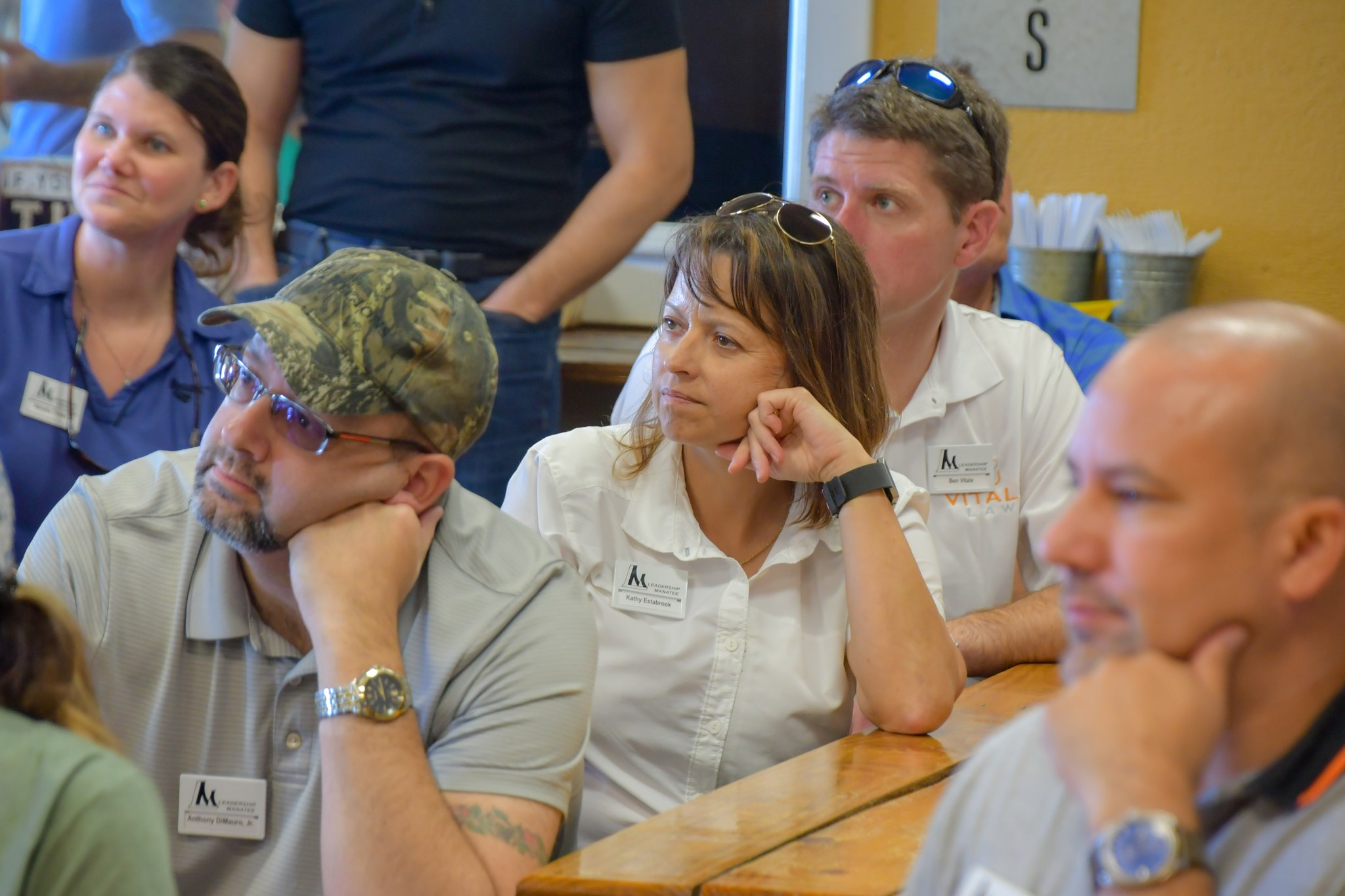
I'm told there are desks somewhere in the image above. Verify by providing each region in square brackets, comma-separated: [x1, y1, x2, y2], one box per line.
[517, 664, 1075, 896]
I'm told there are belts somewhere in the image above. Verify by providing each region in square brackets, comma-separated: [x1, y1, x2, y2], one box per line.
[375, 243, 528, 283]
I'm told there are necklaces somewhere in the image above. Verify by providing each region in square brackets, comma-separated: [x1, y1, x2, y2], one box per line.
[73, 258, 175, 387]
[738, 481, 795, 568]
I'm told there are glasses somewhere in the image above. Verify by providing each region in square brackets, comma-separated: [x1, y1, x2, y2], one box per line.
[208, 340, 432, 457]
[833, 57, 988, 147]
[715, 190, 842, 298]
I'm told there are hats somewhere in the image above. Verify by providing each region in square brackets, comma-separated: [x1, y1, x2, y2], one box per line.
[195, 241, 502, 465]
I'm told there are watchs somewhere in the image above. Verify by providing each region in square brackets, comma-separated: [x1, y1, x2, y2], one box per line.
[315, 665, 415, 724]
[821, 455, 899, 519]
[1087, 807, 1214, 893]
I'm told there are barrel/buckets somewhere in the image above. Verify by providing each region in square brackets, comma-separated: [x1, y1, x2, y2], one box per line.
[1099, 247, 1200, 326]
[998, 245, 1097, 302]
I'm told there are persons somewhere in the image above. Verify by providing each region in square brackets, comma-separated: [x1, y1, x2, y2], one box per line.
[0, 0, 234, 281]
[2, 543, 182, 896]
[610, 57, 1101, 733]
[496, 190, 970, 847]
[1, 42, 256, 592]
[214, 0, 694, 519]
[12, 246, 600, 896]
[889, 299, 1345, 895]
